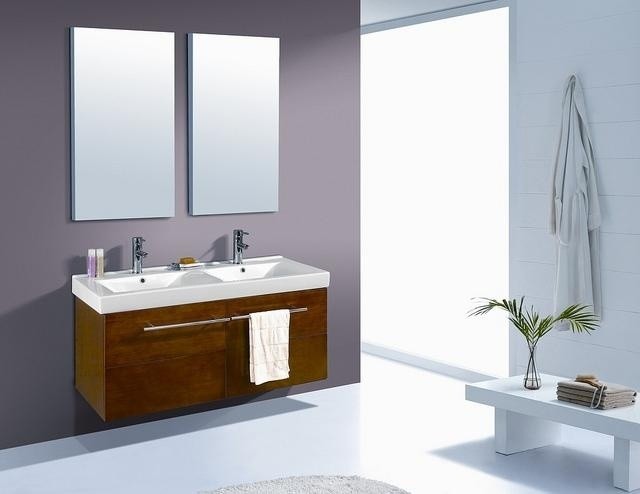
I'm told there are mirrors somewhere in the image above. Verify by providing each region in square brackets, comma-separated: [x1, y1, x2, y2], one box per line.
[188, 32, 280, 217]
[70, 27, 176, 222]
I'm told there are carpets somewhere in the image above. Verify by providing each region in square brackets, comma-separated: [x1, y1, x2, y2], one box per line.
[196, 475, 411, 494]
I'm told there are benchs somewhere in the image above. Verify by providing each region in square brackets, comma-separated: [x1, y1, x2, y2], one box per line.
[465, 373, 640, 492]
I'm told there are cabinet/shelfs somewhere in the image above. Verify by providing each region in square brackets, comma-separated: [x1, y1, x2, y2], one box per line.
[226, 287, 327, 398]
[104, 300, 231, 420]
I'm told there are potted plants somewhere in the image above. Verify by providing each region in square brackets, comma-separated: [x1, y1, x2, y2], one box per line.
[466, 296, 600, 390]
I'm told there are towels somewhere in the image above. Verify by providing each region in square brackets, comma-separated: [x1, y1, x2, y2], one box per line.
[248, 309, 290, 387]
[556, 381, 638, 410]
